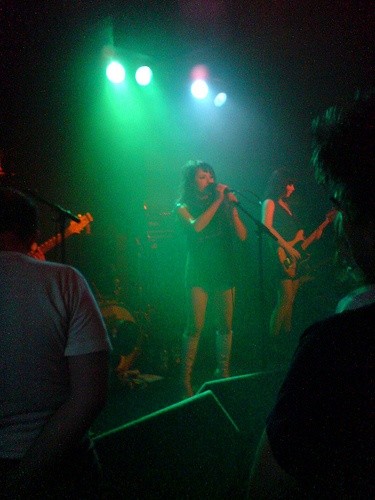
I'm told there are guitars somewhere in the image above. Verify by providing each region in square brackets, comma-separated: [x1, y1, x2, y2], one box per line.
[275, 208, 337, 278]
[25, 212, 93, 262]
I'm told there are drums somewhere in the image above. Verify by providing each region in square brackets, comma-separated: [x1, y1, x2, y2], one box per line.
[97, 303, 138, 373]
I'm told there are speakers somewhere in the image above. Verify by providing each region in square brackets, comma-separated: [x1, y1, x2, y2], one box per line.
[195, 369, 289, 450]
[90, 390, 255, 500]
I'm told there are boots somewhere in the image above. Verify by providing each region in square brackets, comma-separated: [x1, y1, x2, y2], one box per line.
[216, 331, 234, 387]
[179, 333, 197, 405]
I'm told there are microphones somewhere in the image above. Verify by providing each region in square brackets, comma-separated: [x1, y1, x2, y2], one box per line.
[206, 183, 233, 194]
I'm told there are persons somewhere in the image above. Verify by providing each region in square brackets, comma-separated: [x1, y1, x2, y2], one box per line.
[0, 184, 110, 500]
[175, 159, 247, 400]
[245, 88, 375, 500]
[261, 170, 323, 362]
